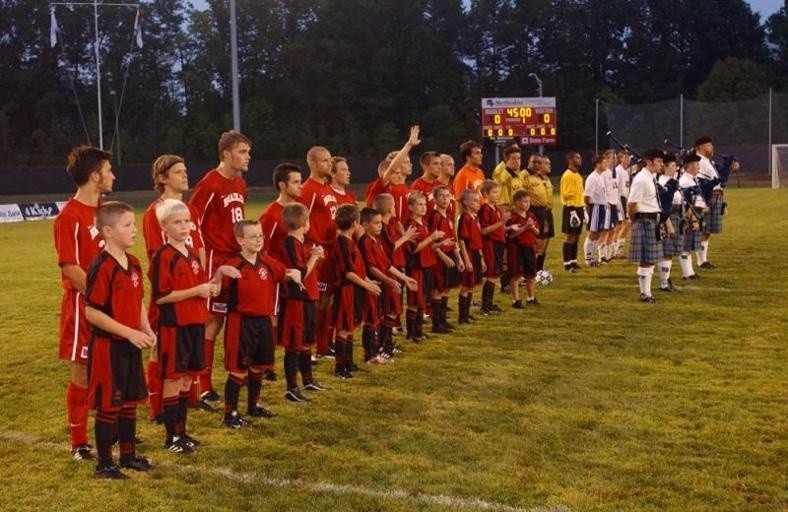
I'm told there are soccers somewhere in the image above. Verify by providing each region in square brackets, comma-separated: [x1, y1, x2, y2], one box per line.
[536, 270, 553, 285]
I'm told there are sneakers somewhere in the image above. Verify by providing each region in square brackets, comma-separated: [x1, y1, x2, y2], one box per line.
[222, 410, 257, 432]
[311, 276, 541, 378]
[283, 387, 312, 404]
[200, 391, 221, 403]
[189, 401, 218, 413]
[94, 463, 130, 482]
[262, 371, 277, 383]
[163, 435, 193, 455]
[247, 406, 277, 418]
[179, 434, 200, 449]
[118, 455, 152, 472]
[565, 254, 715, 305]
[303, 380, 330, 393]
[68, 444, 96, 464]
[149, 415, 166, 427]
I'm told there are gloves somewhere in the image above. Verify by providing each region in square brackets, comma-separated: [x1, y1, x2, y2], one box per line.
[583, 208, 590, 225]
[569, 210, 582, 228]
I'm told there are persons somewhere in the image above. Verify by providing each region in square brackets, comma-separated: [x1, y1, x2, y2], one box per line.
[559, 136, 740, 301]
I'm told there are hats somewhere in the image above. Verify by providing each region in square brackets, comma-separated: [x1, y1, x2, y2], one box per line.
[694, 136, 712, 147]
[640, 150, 677, 163]
[683, 154, 701, 163]
